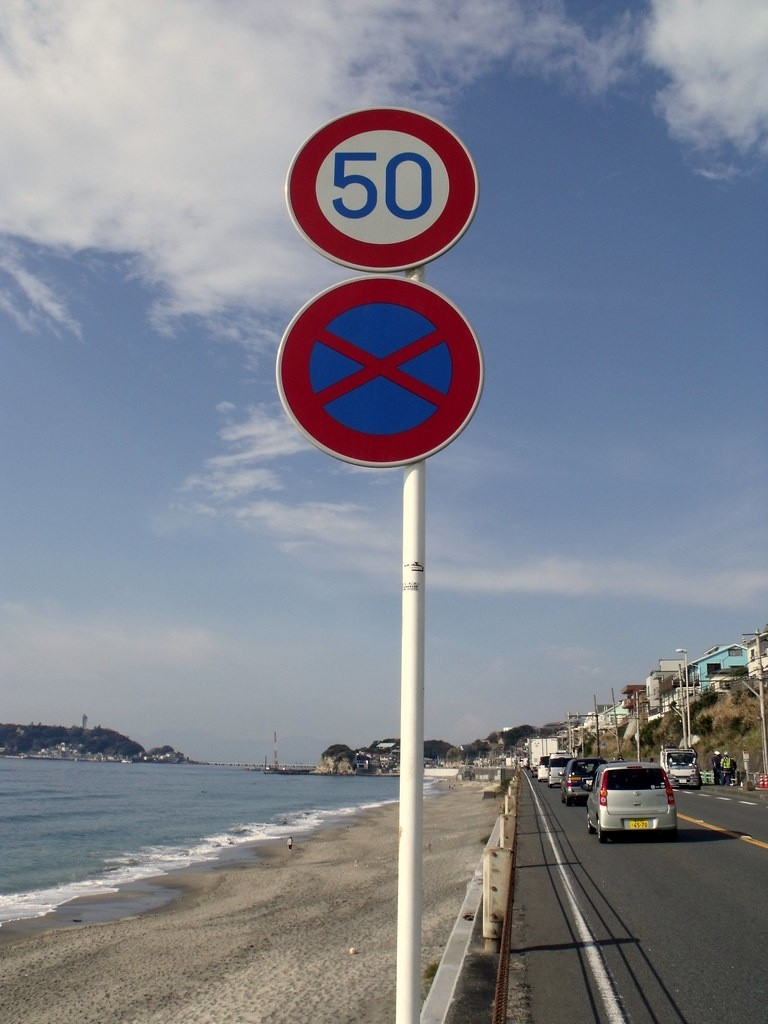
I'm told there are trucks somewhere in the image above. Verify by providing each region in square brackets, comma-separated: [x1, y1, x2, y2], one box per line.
[529, 736, 559, 778]
[660, 747, 701, 790]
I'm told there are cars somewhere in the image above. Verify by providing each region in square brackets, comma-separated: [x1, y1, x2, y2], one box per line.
[581, 762, 678, 844]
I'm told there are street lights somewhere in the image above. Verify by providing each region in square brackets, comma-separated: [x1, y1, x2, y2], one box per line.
[674, 648, 694, 749]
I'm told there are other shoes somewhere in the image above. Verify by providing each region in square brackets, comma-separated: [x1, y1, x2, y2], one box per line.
[714, 783, 721, 785]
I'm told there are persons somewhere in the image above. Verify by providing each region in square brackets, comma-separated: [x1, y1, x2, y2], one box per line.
[711, 751, 737, 786]
[649, 758, 654, 763]
[617, 755, 624, 761]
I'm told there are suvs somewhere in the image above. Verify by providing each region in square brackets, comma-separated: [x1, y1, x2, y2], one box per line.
[548, 751, 574, 789]
[557, 757, 608, 806]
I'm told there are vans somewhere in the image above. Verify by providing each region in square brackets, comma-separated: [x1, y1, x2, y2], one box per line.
[538, 756, 550, 782]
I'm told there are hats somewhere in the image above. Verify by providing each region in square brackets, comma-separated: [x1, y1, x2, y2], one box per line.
[723, 751, 728, 755]
[714, 750, 720, 755]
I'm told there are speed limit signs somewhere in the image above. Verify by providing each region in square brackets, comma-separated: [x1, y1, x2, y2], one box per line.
[285, 105, 482, 275]
